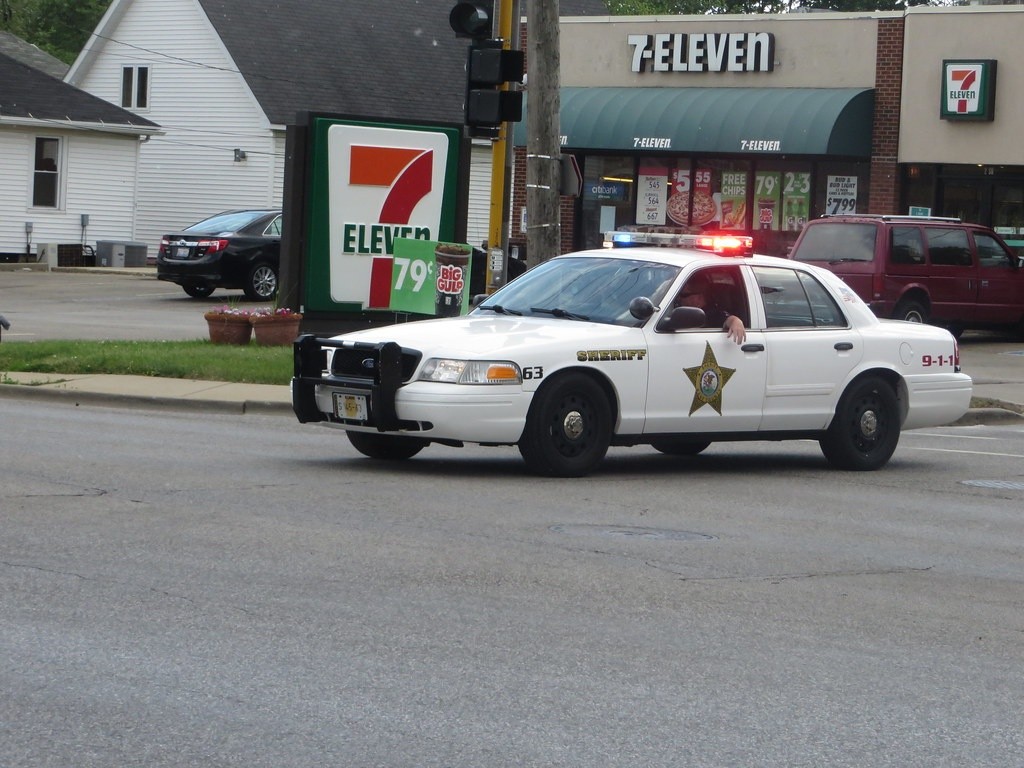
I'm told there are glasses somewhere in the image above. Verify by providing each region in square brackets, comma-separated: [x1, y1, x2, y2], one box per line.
[679, 292, 702, 297]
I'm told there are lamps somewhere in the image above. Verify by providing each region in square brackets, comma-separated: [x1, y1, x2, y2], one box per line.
[233, 149, 246, 161]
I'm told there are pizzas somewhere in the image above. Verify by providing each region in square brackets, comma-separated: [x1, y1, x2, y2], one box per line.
[668, 190, 716, 224]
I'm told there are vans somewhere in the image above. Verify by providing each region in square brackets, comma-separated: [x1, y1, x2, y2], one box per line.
[788, 212, 1024, 343]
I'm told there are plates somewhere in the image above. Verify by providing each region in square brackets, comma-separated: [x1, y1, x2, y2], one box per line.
[666, 211, 716, 226]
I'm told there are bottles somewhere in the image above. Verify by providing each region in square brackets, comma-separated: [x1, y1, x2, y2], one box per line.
[786, 200, 795, 230]
[758, 201, 775, 231]
[797, 199, 807, 231]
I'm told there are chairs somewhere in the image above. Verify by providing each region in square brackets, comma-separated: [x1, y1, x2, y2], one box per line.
[710, 283, 748, 329]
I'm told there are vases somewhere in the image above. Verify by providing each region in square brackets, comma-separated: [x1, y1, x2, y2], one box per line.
[249, 314, 303, 347]
[204, 312, 252, 344]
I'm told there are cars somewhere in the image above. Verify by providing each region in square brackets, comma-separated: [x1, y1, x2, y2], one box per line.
[288, 233, 974, 479]
[157, 207, 282, 302]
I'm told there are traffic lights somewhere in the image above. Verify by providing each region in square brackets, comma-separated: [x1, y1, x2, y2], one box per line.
[447, 0, 494, 42]
[466, 39, 525, 140]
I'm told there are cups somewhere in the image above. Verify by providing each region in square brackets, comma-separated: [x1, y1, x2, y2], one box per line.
[722, 199, 733, 223]
[434, 251, 470, 318]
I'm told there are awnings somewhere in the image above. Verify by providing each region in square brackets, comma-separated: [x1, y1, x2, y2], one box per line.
[512, 84, 874, 161]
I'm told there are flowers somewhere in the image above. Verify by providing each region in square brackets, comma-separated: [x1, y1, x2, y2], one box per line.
[208, 290, 247, 315]
[249, 289, 296, 317]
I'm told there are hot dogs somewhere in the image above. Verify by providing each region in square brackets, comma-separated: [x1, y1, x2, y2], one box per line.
[735, 203, 746, 224]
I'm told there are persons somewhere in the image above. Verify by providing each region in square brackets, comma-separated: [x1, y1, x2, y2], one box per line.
[669, 268, 749, 345]
